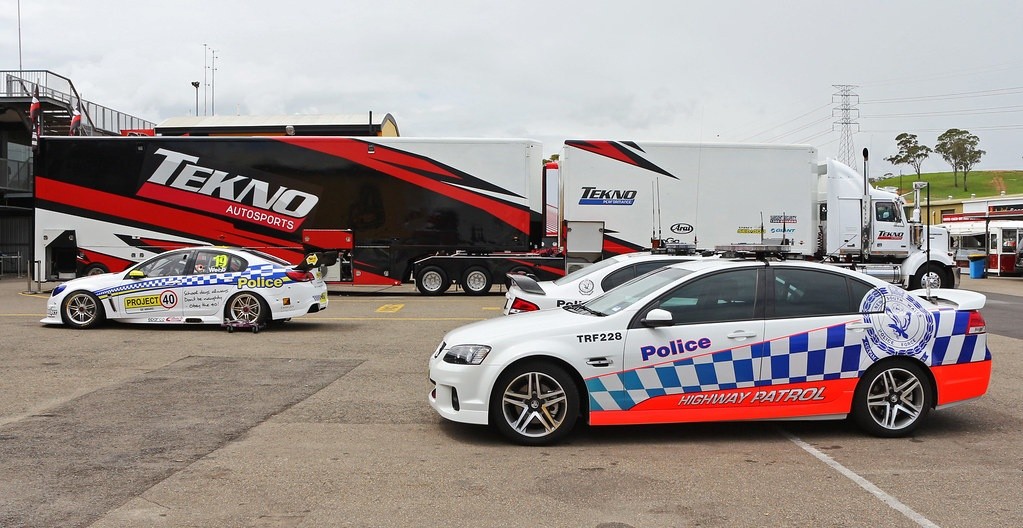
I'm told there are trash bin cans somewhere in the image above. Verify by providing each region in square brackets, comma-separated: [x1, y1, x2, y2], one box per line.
[966, 253, 989, 279]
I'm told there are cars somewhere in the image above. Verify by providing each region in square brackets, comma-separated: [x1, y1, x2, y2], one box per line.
[502, 246, 805, 321]
[424, 240, 991, 446]
[42, 241, 338, 328]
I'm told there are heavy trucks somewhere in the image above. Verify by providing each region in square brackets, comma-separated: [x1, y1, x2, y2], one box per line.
[28, 129, 562, 294]
[554, 139, 958, 293]
